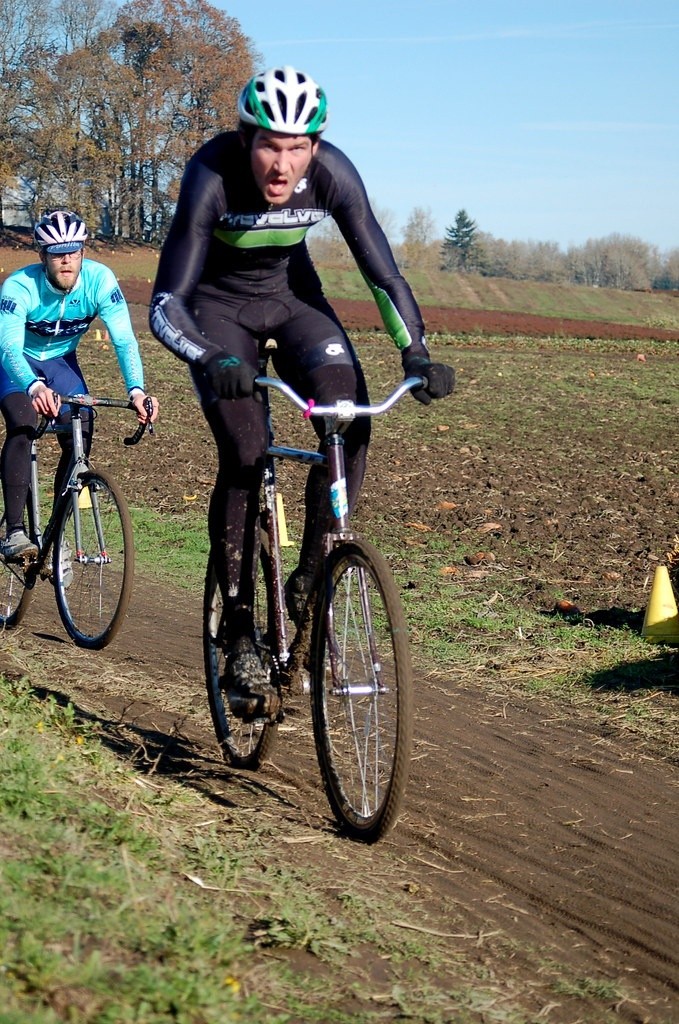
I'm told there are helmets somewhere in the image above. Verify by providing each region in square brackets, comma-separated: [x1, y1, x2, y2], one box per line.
[33, 209, 89, 253]
[237, 67, 328, 135]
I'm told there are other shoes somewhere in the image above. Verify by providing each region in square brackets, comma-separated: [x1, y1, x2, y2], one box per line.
[222, 639, 280, 718]
[286, 567, 316, 625]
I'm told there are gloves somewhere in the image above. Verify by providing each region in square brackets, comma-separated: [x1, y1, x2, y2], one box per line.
[199, 346, 258, 402]
[400, 354, 454, 405]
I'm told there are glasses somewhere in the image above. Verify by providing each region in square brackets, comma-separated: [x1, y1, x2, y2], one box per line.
[48, 251, 82, 261]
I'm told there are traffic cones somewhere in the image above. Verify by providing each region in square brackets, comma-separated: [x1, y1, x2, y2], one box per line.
[639, 566, 679, 642]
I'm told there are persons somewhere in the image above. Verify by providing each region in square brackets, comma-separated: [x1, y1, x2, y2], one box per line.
[147, 65, 454, 718]
[0, 212, 159, 562]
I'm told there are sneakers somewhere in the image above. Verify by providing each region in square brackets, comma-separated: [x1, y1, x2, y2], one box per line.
[46, 540, 74, 588]
[0, 529, 38, 558]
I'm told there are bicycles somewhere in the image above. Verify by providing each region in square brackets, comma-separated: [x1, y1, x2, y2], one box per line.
[205, 339, 429, 847]
[0, 377, 158, 651]
